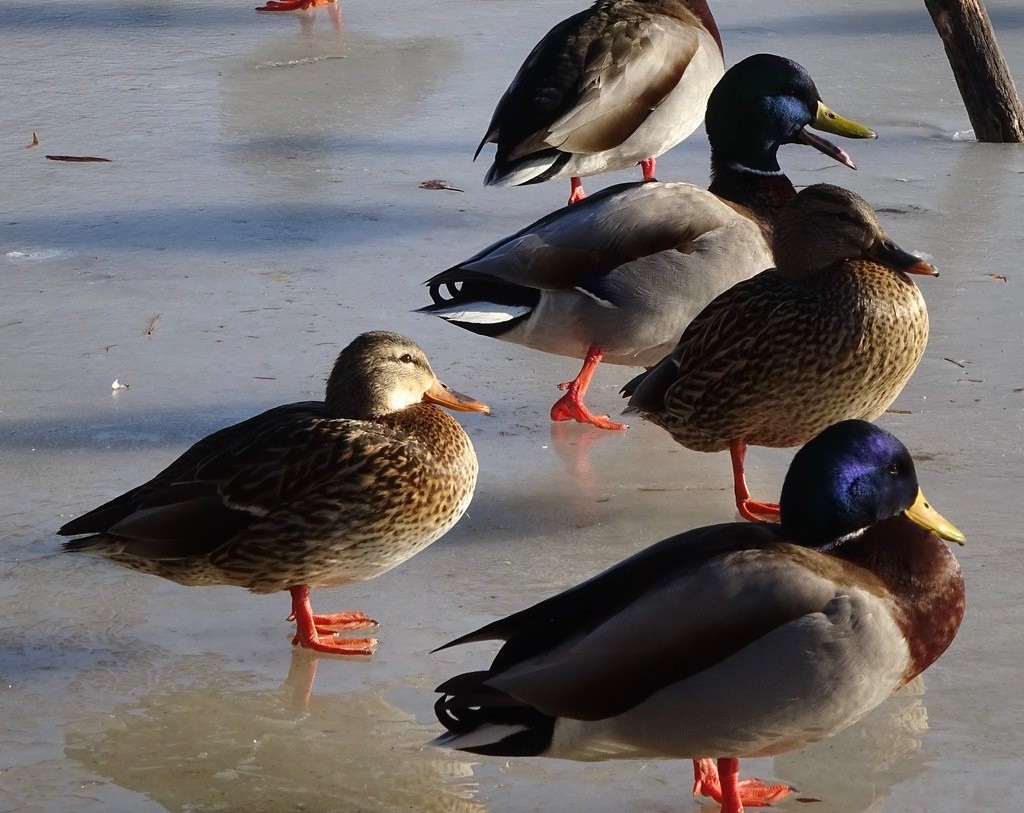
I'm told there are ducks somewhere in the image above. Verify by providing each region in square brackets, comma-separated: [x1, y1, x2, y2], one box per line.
[56, 329, 491, 660]
[408, 1, 968, 813]
